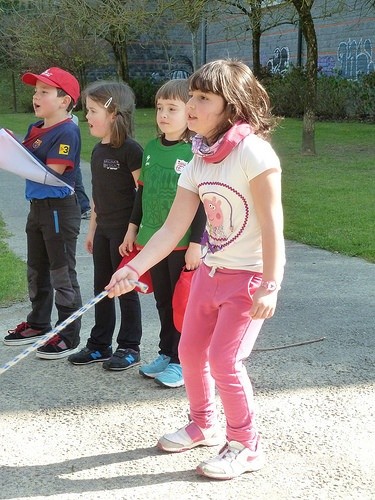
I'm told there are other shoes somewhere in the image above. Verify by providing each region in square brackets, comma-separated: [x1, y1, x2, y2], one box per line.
[81, 209, 93, 220]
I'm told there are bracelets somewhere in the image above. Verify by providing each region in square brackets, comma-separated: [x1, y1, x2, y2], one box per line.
[125, 264, 140, 278]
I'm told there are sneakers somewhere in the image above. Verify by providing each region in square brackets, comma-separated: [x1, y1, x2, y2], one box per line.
[154, 363, 185, 387]
[138, 354, 171, 379]
[36, 334, 80, 359]
[67, 344, 113, 365]
[156, 422, 222, 453]
[101, 347, 142, 371]
[2, 322, 53, 346]
[195, 440, 265, 480]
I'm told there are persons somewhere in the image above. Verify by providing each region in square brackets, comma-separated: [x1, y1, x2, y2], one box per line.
[68, 82, 145, 370]
[2, 66, 91, 359]
[104, 60, 285, 480]
[119, 79, 208, 388]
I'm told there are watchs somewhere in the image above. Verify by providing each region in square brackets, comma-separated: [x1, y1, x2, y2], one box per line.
[258, 279, 282, 291]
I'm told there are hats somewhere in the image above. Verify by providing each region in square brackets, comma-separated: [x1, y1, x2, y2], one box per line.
[21, 67, 81, 107]
[117, 247, 155, 294]
[171, 265, 197, 334]
